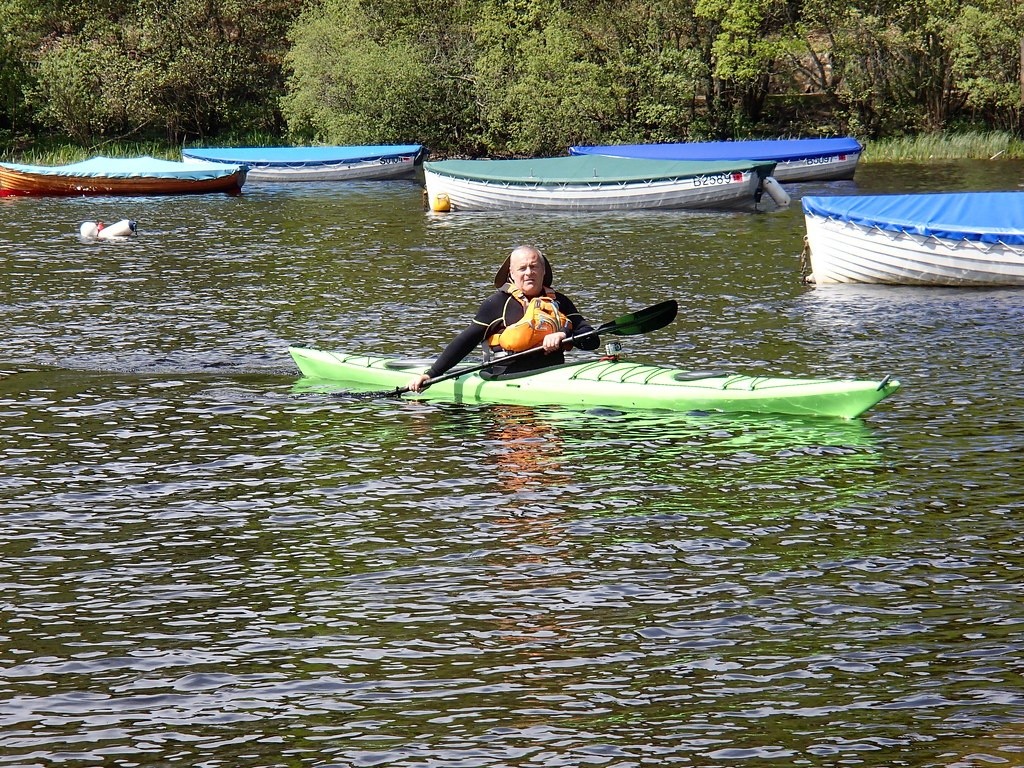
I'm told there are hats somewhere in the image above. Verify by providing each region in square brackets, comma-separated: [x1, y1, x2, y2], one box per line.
[494, 252, 553, 289]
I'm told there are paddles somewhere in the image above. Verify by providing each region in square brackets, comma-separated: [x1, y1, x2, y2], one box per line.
[335, 299, 678, 400]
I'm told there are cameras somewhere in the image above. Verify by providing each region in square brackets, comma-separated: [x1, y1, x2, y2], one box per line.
[605, 341, 623, 356]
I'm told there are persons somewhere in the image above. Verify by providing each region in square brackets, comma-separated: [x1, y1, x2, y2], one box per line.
[406, 244, 600, 392]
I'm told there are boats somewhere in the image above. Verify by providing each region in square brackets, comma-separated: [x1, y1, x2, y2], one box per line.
[570, 138, 863, 185]
[0, 156, 250, 197]
[287, 346, 901, 420]
[423, 155, 791, 211]
[182, 145, 425, 182]
[802, 192, 1024, 287]
